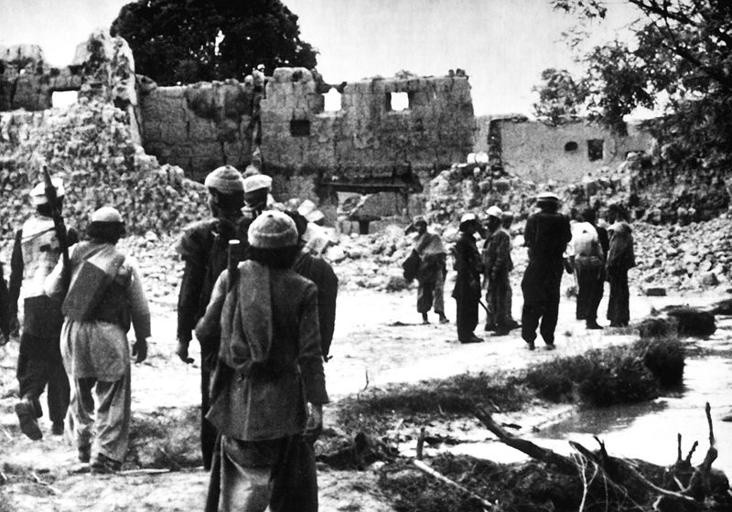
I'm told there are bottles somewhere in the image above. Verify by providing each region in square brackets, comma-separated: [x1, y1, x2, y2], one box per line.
[412, 192, 562, 224]
[244, 175, 273, 193]
[206, 165, 245, 191]
[30, 177, 65, 205]
[93, 207, 124, 222]
[247, 211, 298, 248]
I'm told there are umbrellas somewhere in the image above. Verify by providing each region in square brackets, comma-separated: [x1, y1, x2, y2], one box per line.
[15, 401, 120, 473]
[459, 322, 555, 350]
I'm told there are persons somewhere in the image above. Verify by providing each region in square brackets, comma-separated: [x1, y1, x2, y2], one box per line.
[2, 178, 78, 441]
[562, 205, 636, 329]
[402, 217, 450, 324]
[45, 206, 151, 473]
[478, 206, 520, 336]
[450, 212, 485, 344]
[175, 165, 339, 509]
[522, 191, 572, 350]
